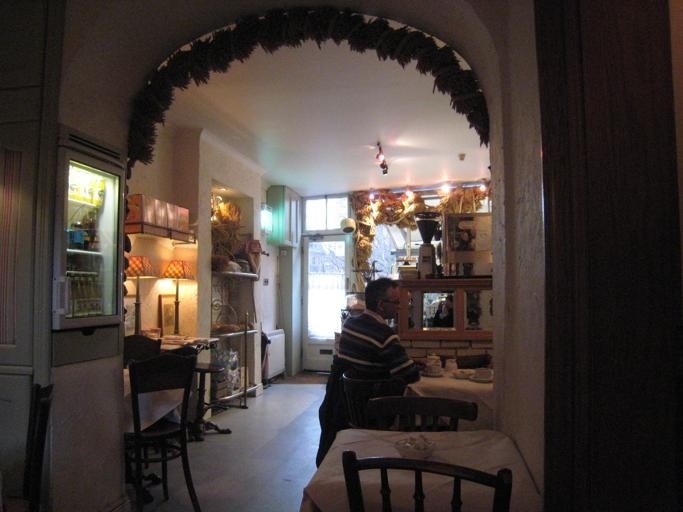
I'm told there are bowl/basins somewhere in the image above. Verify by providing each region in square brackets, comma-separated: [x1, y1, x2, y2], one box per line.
[395, 438, 436, 462]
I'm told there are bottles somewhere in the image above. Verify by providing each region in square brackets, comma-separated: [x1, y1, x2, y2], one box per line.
[64, 270, 104, 320]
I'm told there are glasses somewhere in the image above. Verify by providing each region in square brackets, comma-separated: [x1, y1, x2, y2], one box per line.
[383, 299, 399, 305]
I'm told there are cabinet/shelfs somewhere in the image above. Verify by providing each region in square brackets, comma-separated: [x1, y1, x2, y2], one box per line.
[126, 193, 196, 246]
[266, 186, 302, 248]
[211, 270, 264, 401]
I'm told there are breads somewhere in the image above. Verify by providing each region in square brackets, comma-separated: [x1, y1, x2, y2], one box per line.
[211, 322, 254, 332]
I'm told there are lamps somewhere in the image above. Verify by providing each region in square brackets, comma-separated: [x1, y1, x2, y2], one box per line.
[125, 255, 197, 337]
[340, 195, 356, 235]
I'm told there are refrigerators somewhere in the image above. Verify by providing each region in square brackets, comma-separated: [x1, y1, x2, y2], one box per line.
[52, 121, 124, 334]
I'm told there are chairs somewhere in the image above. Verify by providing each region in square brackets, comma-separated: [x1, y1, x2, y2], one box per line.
[304, 354, 540, 511]
[123, 334, 231, 512]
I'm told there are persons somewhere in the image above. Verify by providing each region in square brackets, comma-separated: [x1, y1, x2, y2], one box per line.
[316, 278, 422, 469]
[432, 295, 454, 327]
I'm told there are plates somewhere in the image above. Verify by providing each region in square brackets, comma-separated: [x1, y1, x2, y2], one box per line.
[421, 366, 494, 382]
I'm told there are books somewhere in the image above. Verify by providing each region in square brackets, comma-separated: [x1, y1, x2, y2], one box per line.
[398, 265, 420, 281]
[162, 335, 209, 346]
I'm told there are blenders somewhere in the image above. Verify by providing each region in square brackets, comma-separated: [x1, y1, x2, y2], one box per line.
[414, 211, 438, 281]
